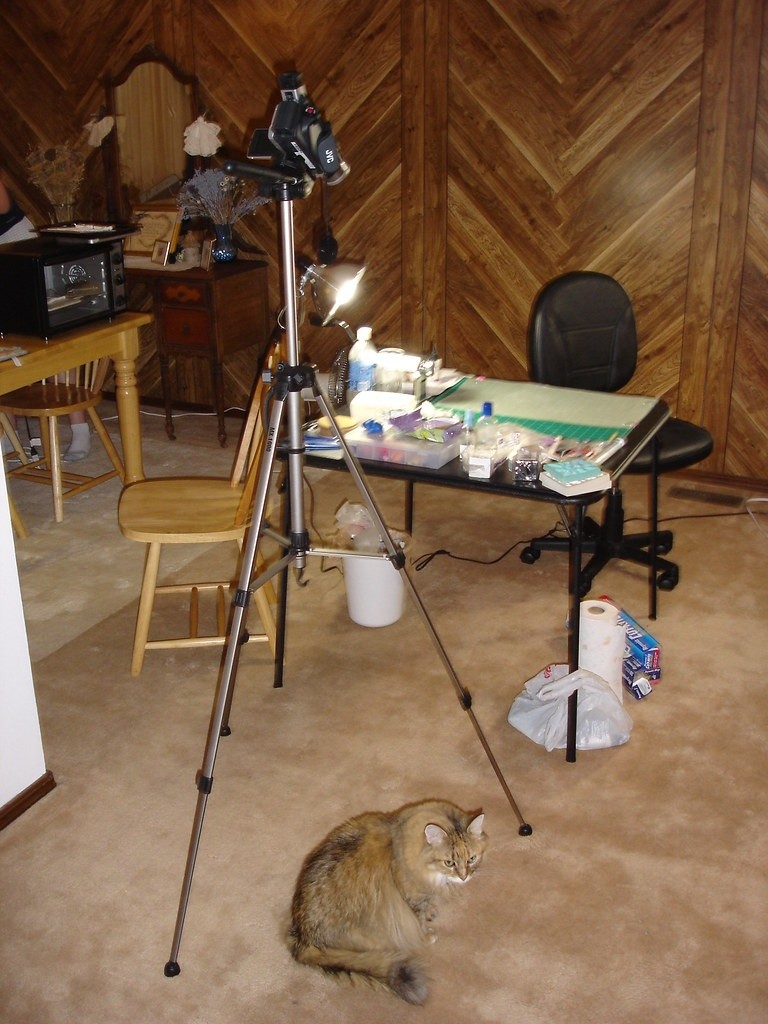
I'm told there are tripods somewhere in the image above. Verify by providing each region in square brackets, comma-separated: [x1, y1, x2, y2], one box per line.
[162, 158, 531, 979]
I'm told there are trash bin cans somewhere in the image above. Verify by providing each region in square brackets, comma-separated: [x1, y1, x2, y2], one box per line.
[332, 529, 415, 627]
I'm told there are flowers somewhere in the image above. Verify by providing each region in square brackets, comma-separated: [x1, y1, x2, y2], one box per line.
[176, 167, 271, 224]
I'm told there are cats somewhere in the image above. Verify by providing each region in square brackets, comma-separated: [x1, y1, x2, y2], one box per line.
[285, 797, 490, 1008]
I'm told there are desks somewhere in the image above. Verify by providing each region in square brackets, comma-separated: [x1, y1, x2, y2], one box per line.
[0, 313, 157, 486]
[273, 371, 671, 763]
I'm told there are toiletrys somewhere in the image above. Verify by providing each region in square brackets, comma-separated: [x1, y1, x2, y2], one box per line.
[594, 596, 663, 702]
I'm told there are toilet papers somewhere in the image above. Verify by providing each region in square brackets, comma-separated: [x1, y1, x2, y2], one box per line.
[578, 600, 627, 708]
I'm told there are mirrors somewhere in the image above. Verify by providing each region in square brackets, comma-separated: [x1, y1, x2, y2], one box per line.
[104, 44, 199, 226]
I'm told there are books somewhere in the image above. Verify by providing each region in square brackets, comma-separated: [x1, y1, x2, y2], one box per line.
[539, 459, 611, 496]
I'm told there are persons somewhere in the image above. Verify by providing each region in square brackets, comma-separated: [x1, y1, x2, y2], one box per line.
[0, 180, 91, 460]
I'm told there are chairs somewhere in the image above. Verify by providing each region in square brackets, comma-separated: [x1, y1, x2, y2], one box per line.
[118, 334, 280, 675]
[0, 357, 125, 523]
[520, 271, 712, 595]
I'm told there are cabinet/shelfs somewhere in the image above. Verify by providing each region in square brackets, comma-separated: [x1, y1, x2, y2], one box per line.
[101, 259, 269, 449]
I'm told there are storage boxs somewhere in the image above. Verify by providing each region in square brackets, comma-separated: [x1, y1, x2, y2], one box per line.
[344, 419, 468, 469]
[514, 460, 538, 481]
[460, 432, 520, 478]
[598, 595, 663, 700]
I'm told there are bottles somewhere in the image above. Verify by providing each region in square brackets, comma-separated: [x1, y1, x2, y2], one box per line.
[474, 402, 499, 459]
[348, 327, 377, 403]
[459, 408, 474, 461]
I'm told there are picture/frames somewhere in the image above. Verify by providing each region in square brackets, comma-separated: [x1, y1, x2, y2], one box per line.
[200, 238, 213, 271]
[122, 205, 185, 257]
[150, 239, 170, 267]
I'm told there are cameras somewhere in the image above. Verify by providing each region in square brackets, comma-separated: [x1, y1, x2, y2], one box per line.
[269, 70, 350, 186]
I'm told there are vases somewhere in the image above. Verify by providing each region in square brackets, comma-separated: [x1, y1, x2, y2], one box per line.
[50, 200, 78, 223]
[212, 224, 237, 264]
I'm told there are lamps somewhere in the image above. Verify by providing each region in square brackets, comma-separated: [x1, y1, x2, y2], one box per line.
[295, 252, 370, 342]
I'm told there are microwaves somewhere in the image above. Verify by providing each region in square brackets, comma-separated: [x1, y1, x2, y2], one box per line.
[0, 237, 127, 340]
